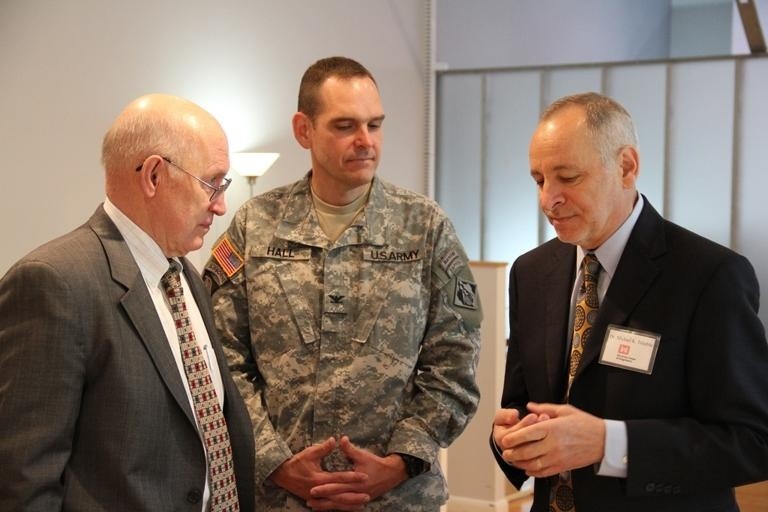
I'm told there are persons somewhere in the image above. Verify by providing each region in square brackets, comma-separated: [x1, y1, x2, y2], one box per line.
[488, 91, 768, 512]
[201, 55, 480, 512]
[0, 90, 257, 512]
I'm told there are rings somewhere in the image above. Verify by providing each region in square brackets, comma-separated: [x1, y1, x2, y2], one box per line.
[535, 458, 542, 472]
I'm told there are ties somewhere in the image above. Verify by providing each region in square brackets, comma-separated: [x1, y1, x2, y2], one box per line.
[548, 253, 601, 511]
[162, 267, 242, 512]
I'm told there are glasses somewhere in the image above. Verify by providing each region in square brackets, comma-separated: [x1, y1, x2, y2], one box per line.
[135, 156, 233, 203]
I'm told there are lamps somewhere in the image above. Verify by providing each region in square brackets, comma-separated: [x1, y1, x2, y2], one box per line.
[230, 153, 279, 199]
